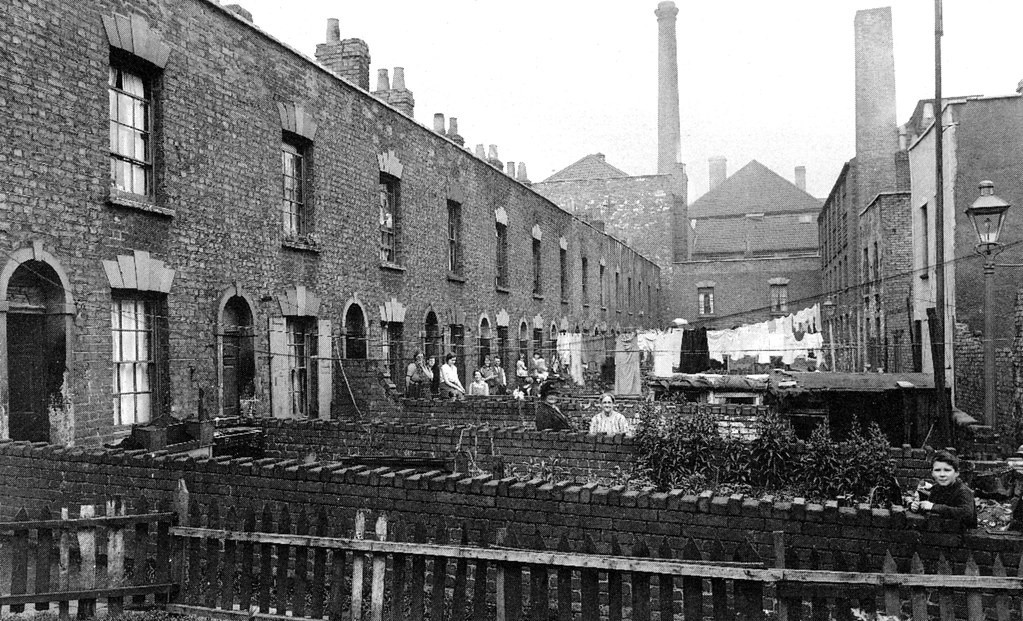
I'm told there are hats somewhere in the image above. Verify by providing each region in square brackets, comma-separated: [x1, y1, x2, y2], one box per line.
[540, 380, 560, 396]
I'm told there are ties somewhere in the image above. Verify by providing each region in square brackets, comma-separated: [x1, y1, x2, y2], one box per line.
[496, 366, 499, 374]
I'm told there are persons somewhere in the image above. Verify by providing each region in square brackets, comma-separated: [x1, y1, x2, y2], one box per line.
[589, 393, 629, 436]
[535, 381, 573, 430]
[406, 350, 587, 401]
[707, 367, 729, 375]
[905, 451, 978, 530]
[640, 346, 654, 367]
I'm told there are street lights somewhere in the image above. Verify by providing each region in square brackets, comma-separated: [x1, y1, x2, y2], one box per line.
[962, 178, 1014, 453]
